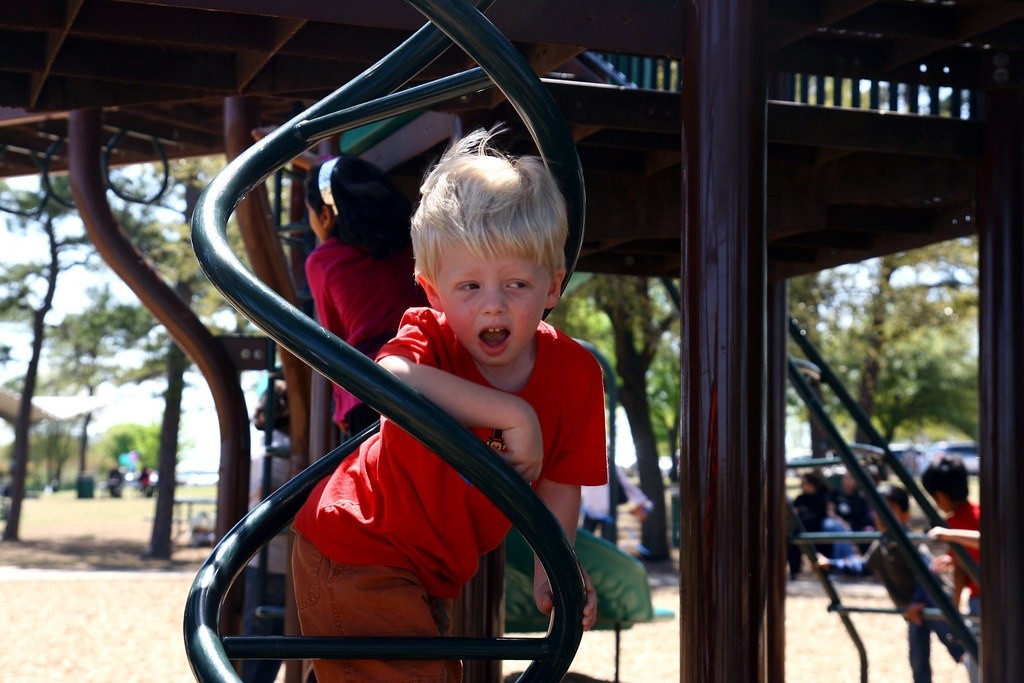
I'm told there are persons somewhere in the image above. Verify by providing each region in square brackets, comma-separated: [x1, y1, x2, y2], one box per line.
[303, 155, 433, 438]
[291, 123, 606, 683]
[578, 456, 653, 544]
[785, 495, 804, 579]
[841, 462, 876, 530]
[138, 468, 159, 496]
[820, 493, 868, 577]
[109, 465, 126, 497]
[793, 473, 824, 533]
[920, 455, 981, 683]
[813, 484, 981, 683]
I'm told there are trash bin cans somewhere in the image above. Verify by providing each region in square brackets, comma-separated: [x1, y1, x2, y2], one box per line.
[77, 476, 94, 498]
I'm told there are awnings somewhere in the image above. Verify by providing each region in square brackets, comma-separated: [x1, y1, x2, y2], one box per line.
[785, 442, 887, 468]
